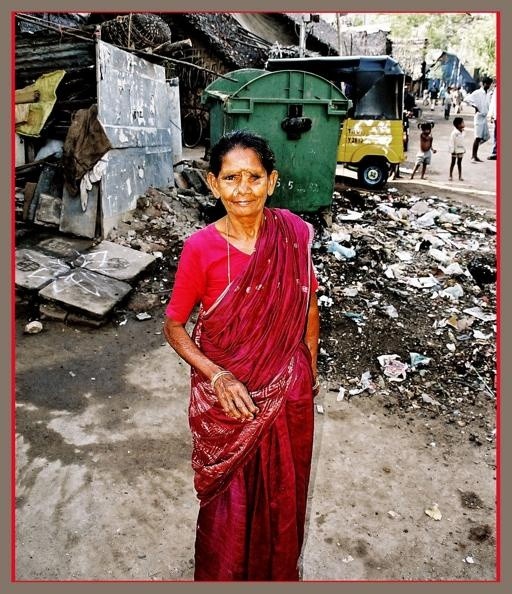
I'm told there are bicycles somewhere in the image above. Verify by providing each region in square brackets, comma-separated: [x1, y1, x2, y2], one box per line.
[182, 96, 209, 148]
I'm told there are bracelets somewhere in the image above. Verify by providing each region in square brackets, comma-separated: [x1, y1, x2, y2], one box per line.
[210, 368, 235, 394]
[312, 380, 320, 391]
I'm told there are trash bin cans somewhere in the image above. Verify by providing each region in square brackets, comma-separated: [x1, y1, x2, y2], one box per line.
[201, 67, 352, 212]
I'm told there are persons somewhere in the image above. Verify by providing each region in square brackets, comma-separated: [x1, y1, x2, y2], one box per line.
[409, 120, 438, 179]
[420, 80, 469, 121]
[487, 85, 497, 160]
[461, 76, 493, 163]
[445, 115, 467, 181]
[160, 127, 321, 587]
[388, 73, 416, 178]
